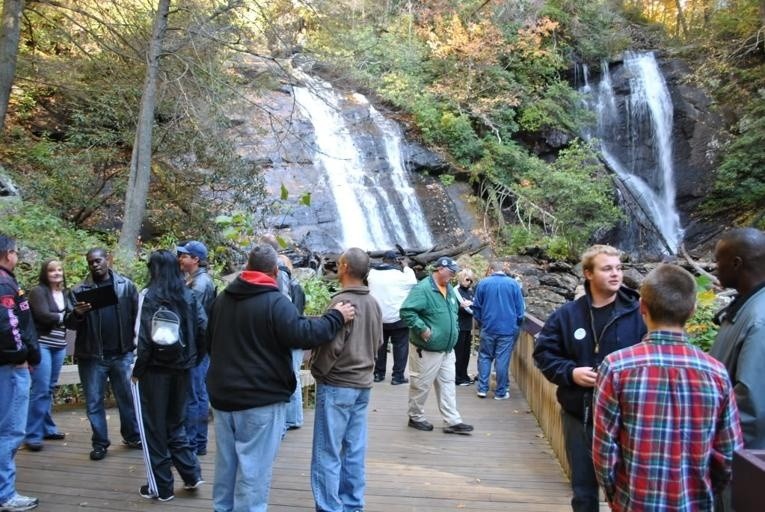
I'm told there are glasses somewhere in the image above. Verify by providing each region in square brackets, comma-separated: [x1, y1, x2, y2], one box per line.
[10, 248, 21, 258]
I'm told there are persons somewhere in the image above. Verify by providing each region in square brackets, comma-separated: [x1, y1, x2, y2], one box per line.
[590, 262, 746, 511]
[533, 245, 649, 511]
[708, 227, 765, 512]
[1, 236, 138, 512]
[368, 252, 525, 434]
[131, 240, 384, 511]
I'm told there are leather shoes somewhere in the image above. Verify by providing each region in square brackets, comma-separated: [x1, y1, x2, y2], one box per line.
[42, 432, 65, 440]
[24, 442, 42, 452]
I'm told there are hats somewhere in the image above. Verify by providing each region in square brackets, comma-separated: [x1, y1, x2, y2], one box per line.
[433, 256, 463, 273]
[175, 240, 209, 262]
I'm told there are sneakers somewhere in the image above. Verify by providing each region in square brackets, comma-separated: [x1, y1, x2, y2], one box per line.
[391, 377, 408, 384]
[123, 440, 143, 450]
[408, 419, 433, 431]
[459, 376, 510, 401]
[89, 445, 107, 461]
[374, 373, 385, 382]
[444, 423, 474, 435]
[182, 475, 206, 489]
[0, 490, 40, 512]
[139, 484, 174, 502]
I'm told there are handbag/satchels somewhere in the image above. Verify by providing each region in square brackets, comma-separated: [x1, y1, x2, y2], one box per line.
[147, 287, 184, 353]
